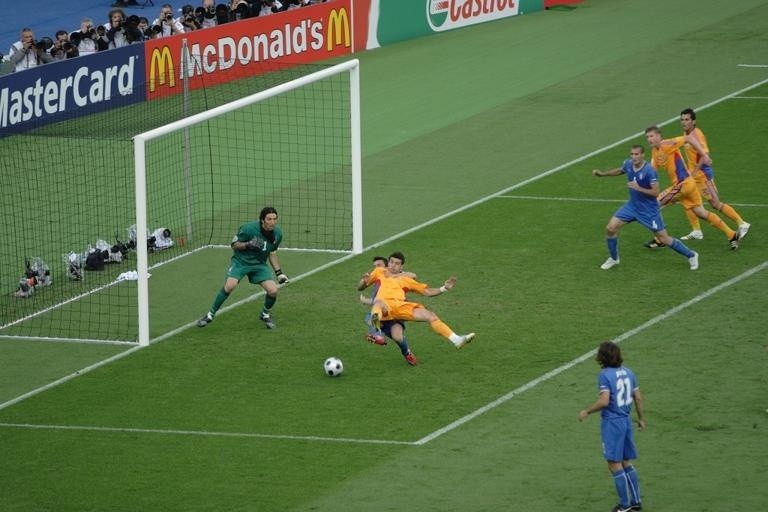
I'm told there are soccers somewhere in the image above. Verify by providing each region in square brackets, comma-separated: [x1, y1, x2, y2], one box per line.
[323, 357, 343, 376]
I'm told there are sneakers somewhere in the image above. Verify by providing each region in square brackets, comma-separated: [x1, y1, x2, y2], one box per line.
[642, 234, 669, 249]
[728, 232, 739, 252]
[679, 230, 704, 241]
[403, 349, 417, 366]
[454, 332, 478, 352]
[687, 250, 700, 271]
[610, 503, 643, 512]
[600, 255, 621, 271]
[737, 222, 751, 241]
[259, 311, 275, 331]
[195, 314, 215, 327]
[365, 313, 388, 348]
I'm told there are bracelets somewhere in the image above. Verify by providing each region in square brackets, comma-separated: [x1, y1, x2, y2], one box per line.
[275, 269, 282, 276]
[440, 286, 448, 293]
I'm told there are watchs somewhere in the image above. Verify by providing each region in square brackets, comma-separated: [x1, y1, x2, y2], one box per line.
[362, 250, 477, 349]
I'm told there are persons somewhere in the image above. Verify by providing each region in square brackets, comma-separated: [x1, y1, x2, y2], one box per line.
[357, 256, 417, 366]
[678, 109, 750, 241]
[592, 146, 699, 271]
[0, 0, 324, 73]
[644, 125, 741, 250]
[196, 207, 290, 330]
[578, 340, 648, 510]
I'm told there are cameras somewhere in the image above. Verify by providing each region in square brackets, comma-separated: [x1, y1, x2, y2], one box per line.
[28, 0, 248, 50]
